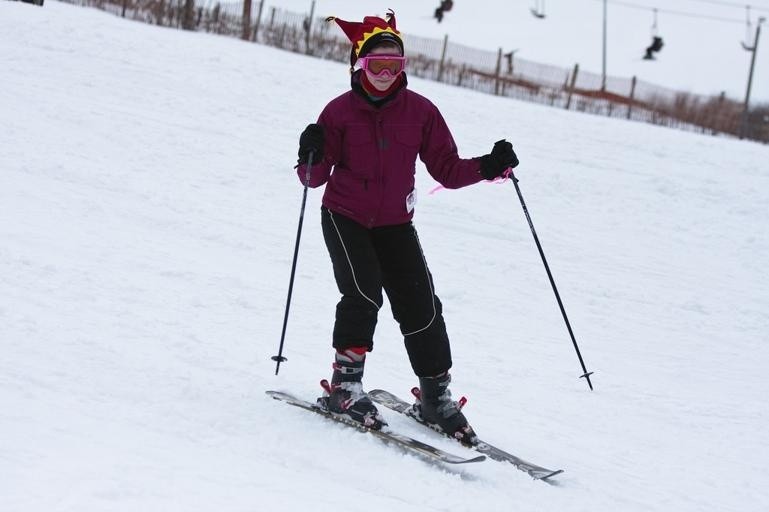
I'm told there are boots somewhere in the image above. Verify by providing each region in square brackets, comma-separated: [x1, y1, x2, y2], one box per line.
[329, 347, 382, 430]
[420, 370, 476, 442]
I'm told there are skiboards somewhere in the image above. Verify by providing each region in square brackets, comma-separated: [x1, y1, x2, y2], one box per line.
[267, 389, 564, 480]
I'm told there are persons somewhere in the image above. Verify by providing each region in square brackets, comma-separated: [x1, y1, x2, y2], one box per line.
[433, 1, 454, 22]
[297, 8, 518, 446]
[643, 37, 660, 58]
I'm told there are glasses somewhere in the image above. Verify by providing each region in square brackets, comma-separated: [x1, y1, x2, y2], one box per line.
[353, 53, 406, 79]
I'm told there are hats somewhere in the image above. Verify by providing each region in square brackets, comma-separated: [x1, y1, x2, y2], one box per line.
[326, 7, 404, 74]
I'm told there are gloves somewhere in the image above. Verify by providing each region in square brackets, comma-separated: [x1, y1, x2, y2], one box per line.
[298, 124, 324, 165]
[480, 142, 519, 180]
